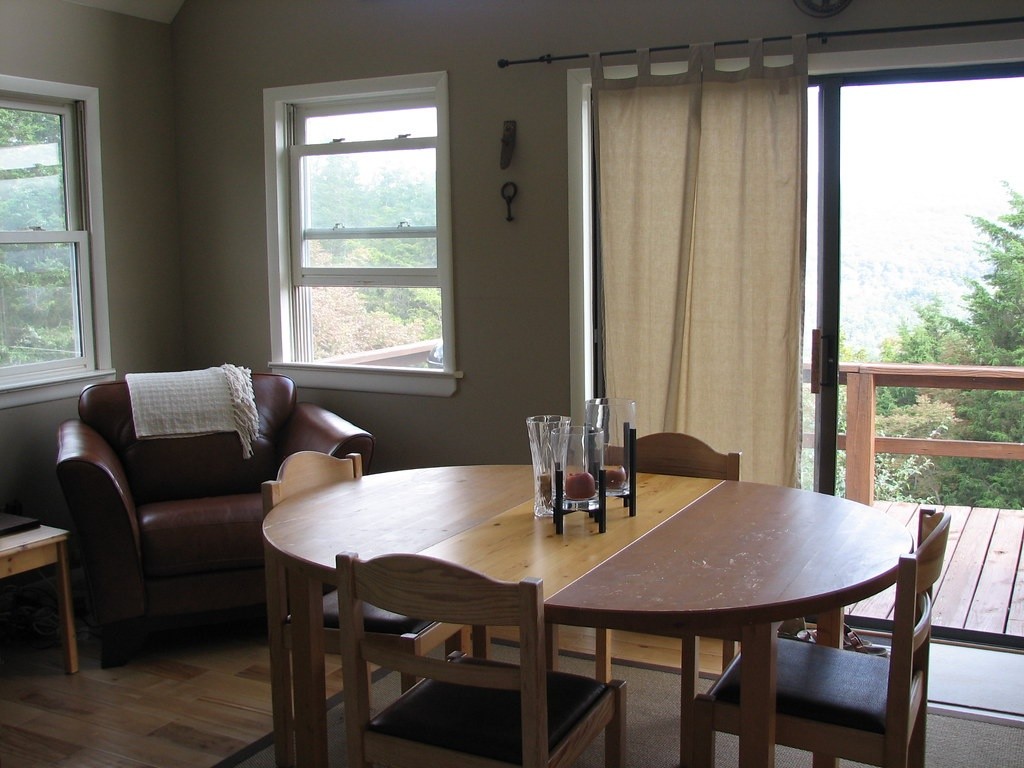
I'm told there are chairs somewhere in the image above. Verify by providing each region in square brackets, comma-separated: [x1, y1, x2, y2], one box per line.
[594, 433, 742, 684]
[262, 451, 471, 768]
[335, 551, 628, 767]
[691, 507, 953, 768]
[54, 372, 374, 670]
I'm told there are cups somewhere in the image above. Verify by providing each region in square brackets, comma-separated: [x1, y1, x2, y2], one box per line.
[584, 397, 637, 496]
[550, 424, 604, 511]
[525, 415, 571, 517]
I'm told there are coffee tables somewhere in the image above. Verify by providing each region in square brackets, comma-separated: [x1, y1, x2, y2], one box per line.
[1, 523, 80, 674]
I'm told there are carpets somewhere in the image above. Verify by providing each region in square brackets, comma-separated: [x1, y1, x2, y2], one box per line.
[211, 636, 1024, 768]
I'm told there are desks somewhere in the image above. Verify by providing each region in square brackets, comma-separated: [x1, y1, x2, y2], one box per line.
[261, 462, 914, 767]
